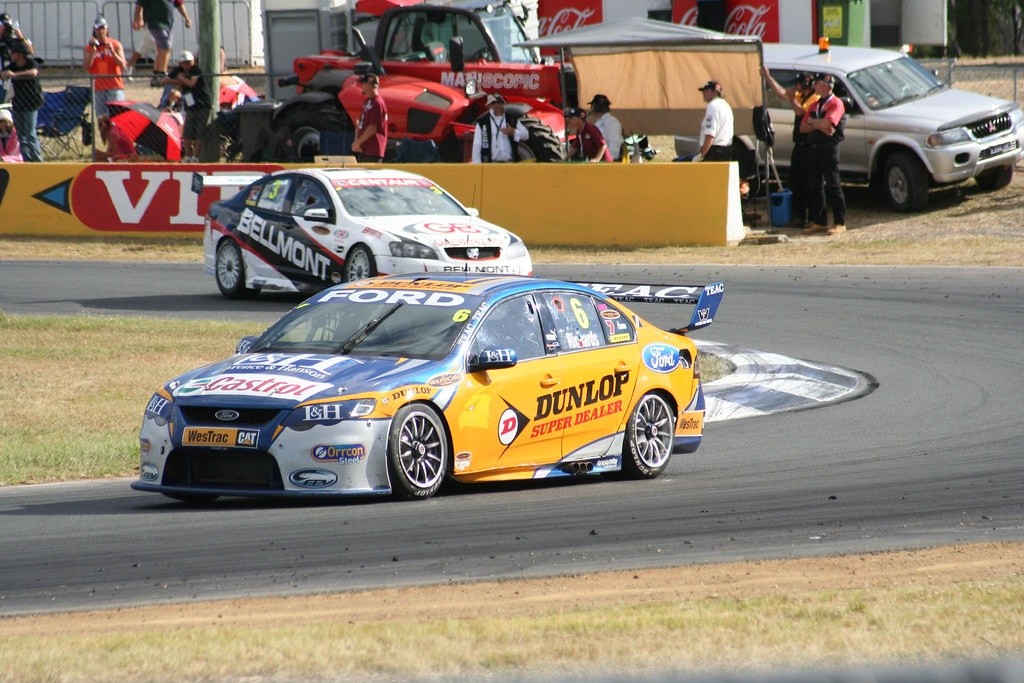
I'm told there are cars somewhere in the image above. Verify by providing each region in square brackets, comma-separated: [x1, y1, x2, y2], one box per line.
[129, 268, 723, 503]
[191, 165, 535, 298]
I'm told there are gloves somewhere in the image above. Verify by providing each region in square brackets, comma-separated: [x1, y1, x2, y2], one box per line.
[691, 153, 703, 163]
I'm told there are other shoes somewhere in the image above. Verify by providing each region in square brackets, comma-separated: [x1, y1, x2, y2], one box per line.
[191, 156, 200, 162]
[151, 74, 160, 86]
[125, 66, 134, 82]
[158, 73, 171, 84]
[180, 156, 190, 163]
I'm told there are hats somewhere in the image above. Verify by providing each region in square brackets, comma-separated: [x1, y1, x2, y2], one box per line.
[587, 95, 610, 106]
[809, 73, 835, 83]
[564, 108, 586, 119]
[179, 50, 194, 64]
[485, 92, 506, 108]
[698, 81, 722, 95]
[357, 73, 380, 86]
[789, 72, 813, 85]
[93, 18, 108, 29]
[0, 109, 13, 123]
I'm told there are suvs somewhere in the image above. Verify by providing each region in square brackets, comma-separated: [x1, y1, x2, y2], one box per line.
[674, 38, 1024, 212]
[270, 1, 580, 163]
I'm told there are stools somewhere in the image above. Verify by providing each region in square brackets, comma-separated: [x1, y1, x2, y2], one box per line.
[742, 174, 766, 227]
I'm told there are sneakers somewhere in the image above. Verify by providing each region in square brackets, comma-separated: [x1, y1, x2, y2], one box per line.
[803, 223, 827, 235]
[827, 224, 847, 236]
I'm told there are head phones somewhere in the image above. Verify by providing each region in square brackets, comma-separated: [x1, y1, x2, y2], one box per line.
[800, 72, 811, 90]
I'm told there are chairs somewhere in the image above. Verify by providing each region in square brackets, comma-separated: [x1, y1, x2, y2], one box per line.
[10, 85, 95, 160]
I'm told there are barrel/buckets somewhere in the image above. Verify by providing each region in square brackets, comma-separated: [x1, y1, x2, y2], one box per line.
[769, 187, 793, 227]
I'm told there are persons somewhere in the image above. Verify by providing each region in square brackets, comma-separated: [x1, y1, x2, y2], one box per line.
[351, 71, 388, 163]
[561, 106, 613, 163]
[469, 93, 529, 163]
[123, 27, 158, 82]
[82, 17, 127, 118]
[694, 79, 734, 162]
[586, 94, 624, 162]
[160, 49, 212, 165]
[132, 0, 192, 86]
[0, 13, 44, 164]
[758, 66, 846, 237]
